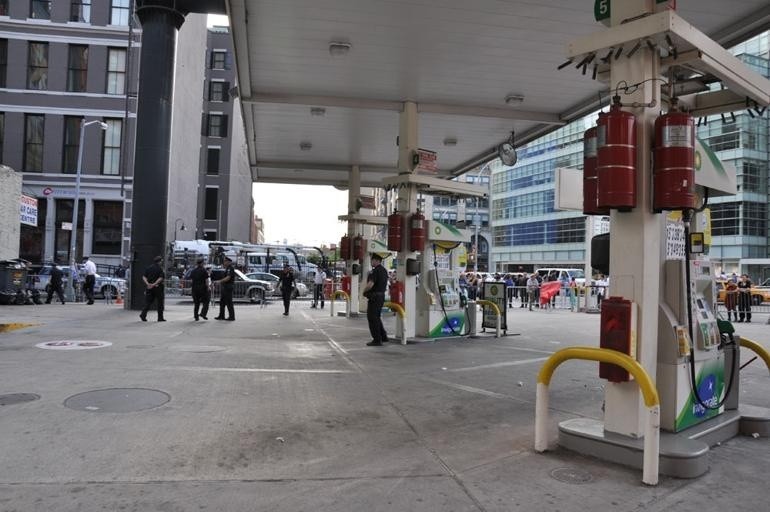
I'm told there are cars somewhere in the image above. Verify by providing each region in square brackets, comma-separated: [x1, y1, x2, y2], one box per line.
[327, 267, 397, 293]
[464, 270, 530, 296]
[245, 273, 308, 300]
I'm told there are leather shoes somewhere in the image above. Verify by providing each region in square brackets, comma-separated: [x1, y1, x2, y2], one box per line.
[214, 316, 225, 320]
[382, 337, 388, 342]
[140, 315, 147, 321]
[225, 318, 235, 321]
[200, 313, 208, 320]
[366, 337, 383, 346]
[158, 318, 166, 321]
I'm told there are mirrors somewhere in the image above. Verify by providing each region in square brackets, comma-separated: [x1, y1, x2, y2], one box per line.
[499, 142, 518, 166]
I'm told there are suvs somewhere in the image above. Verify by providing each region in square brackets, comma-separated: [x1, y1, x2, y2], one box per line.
[175, 266, 274, 303]
[32, 265, 127, 300]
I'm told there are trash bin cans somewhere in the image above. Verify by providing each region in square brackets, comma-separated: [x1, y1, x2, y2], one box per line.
[0, 258, 32, 305]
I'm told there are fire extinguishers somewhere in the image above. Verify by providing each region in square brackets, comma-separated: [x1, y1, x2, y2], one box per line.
[354, 233, 363, 259]
[654, 98, 696, 208]
[584, 112, 611, 214]
[388, 208, 401, 251]
[341, 234, 349, 259]
[596, 95, 637, 207]
[411, 208, 426, 251]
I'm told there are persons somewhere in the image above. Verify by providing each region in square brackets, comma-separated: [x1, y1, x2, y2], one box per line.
[309, 265, 327, 310]
[213, 257, 235, 321]
[274, 262, 296, 316]
[738, 273, 752, 323]
[460, 265, 608, 314]
[71, 261, 80, 302]
[139, 256, 167, 322]
[82, 257, 96, 305]
[44, 263, 66, 305]
[723, 277, 739, 323]
[362, 252, 388, 346]
[191, 258, 212, 321]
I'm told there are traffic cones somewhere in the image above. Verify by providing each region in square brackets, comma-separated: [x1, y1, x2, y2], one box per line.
[116, 289, 123, 304]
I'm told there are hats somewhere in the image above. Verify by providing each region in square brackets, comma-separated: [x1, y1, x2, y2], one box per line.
[223, 256, 232, 263]
[372, 253, 383, 261]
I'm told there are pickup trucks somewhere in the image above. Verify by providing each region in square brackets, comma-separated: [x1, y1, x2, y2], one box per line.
[715, 278, 770, 306]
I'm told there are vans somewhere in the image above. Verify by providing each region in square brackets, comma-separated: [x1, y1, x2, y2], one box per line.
[530, 267, 595, 294]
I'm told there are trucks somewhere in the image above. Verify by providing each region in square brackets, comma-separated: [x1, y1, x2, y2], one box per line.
[278, 252, 318, 278]
[174, 240, 281, 284]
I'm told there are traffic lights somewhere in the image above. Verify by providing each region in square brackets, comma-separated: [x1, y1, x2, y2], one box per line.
[468, 254, 475, 262]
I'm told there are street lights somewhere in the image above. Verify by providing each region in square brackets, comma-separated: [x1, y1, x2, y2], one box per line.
[442, 203, 465, 224]
[173, 218, 188, 241]
[473, 158, 520, 283]
[60, 119, 108, 306]
